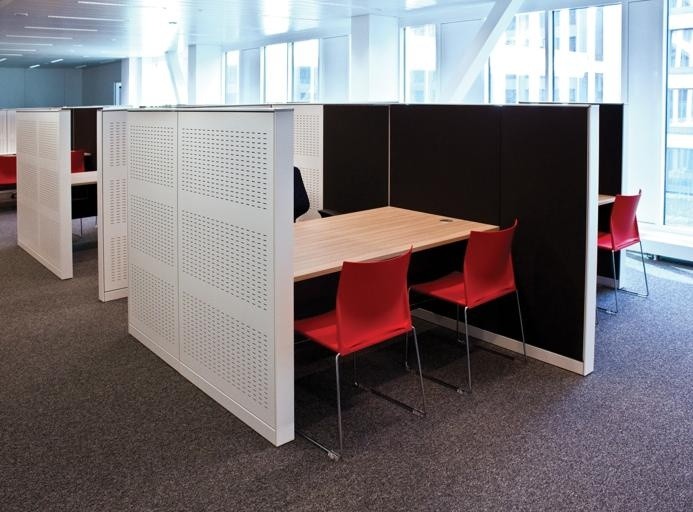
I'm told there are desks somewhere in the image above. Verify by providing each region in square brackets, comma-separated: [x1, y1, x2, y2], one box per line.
[290, 207, 502, 287]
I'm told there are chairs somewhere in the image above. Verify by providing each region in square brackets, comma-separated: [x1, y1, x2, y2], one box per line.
[296, 255, 426, 457]
[595, 190, 648, 315]
[403, 218, 521, 397]
